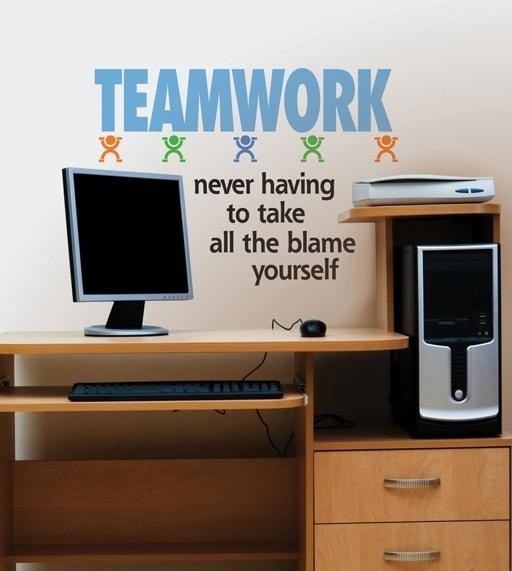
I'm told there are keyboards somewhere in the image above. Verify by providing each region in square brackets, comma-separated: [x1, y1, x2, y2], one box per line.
[68, 381, 284, 399]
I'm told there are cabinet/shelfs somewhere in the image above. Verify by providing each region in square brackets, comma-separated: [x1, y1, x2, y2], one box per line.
[0, 203, 512, 568]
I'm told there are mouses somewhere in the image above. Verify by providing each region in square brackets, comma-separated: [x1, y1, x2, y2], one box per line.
[300, 320, 326, 337]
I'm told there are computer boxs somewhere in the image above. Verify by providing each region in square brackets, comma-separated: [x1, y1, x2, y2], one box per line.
[391, 242, 501, 438]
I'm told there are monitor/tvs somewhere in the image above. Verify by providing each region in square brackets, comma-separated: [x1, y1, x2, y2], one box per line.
[62, 167, 195, 336]
